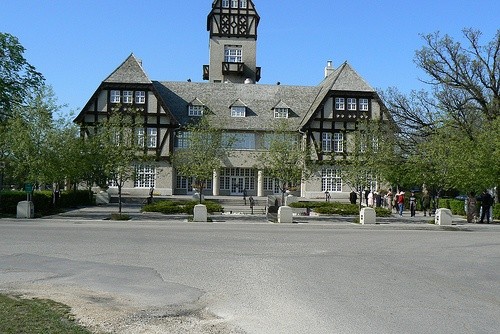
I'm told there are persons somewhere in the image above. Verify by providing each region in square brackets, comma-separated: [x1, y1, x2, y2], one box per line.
[350, 190, 357, 204]
[409, 192, 417, 217]
[365, 188, 405, 217]
[476, 189, 493, 224]
[423, 190, 431, 216]
[243, 188, 246, 205]
[249, 195, 253, 214]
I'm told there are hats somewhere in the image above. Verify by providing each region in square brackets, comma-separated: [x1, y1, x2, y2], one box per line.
[399, 191, 405, 195]
